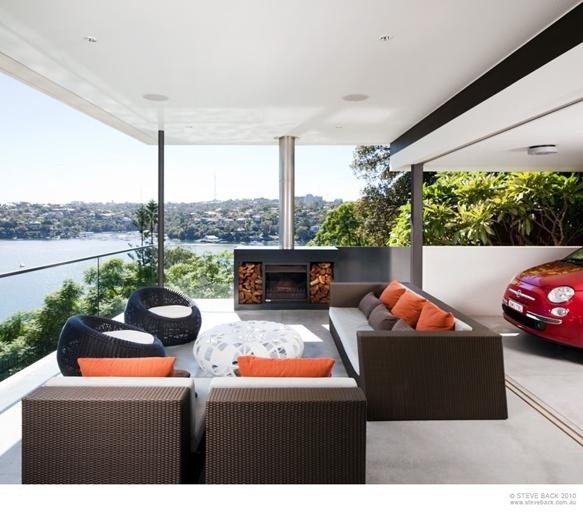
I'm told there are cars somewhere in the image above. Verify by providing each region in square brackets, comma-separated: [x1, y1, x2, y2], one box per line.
[500, 247, 582, 351]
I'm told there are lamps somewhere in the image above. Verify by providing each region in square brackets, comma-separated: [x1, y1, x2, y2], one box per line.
[528, 144, 558, 156]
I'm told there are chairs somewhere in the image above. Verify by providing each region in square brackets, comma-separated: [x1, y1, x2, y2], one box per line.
[21, 378, 206, 484]
[206, 377, 368, 484]
[124, 288, 202, 345]
[56, 315, 166, 377]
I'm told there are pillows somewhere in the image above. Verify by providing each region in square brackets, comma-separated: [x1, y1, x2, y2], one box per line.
[378, 281, 406, 311]
[237, 356, 335, 377]
[391, 289, 426, 328]
[367, 303, 398, 331]
[391, 318, 416, 331]
[77, 357, 176, 377]
[358, 292, 382, 321]
[416, 300, 455, 332]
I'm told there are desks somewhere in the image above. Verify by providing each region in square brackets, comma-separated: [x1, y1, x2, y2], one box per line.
[193, 320, 305, 376]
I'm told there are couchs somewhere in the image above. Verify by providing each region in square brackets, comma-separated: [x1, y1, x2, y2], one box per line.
[328, 281, 509, 421]
